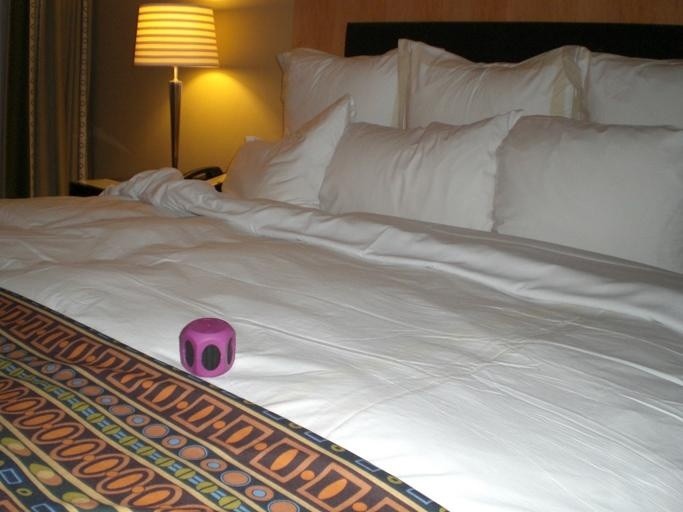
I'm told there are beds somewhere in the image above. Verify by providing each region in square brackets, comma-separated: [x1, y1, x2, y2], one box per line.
[0, 19, 683, 512]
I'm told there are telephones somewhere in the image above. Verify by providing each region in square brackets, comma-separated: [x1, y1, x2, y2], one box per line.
[183, 165, 221, 180]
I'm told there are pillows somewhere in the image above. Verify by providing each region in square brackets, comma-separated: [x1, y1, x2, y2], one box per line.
[221, 93, 357, 209]
[493, 114, 682, 274]
[583, 51, 683, 126]
[318, 108, 526, 234]
[397, 38, 592, 130]
[274, 47, 398, 135]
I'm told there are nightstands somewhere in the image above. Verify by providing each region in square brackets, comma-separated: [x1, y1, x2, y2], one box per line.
[69, 173, 227, 197]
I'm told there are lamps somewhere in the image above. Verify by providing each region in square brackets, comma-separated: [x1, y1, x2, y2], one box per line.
[134, 3, 220, 169]
